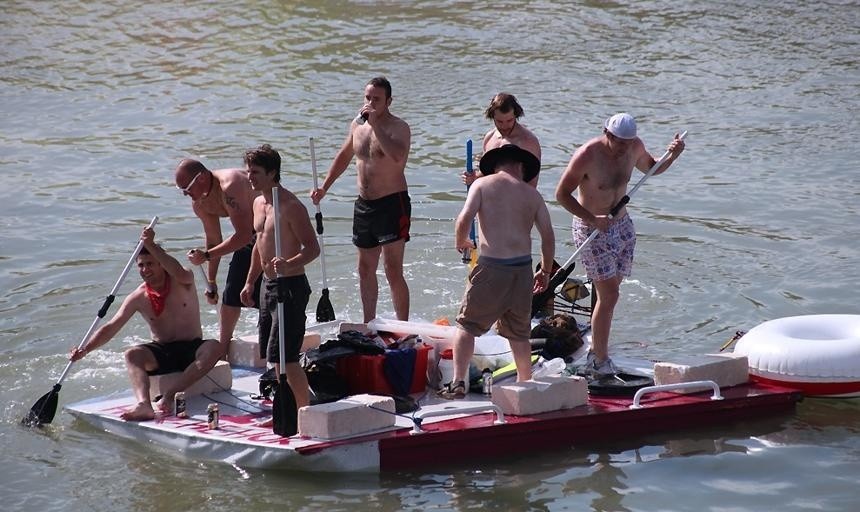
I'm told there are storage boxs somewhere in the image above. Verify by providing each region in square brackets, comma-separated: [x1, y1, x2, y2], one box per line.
[336, 343, 433, 395]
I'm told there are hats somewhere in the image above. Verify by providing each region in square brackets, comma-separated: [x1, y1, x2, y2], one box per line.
[605, 112, 637, 139]
[479, 144, 541, 183]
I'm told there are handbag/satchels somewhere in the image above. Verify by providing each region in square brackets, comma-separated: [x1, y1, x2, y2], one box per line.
[531, 314, 584, 362]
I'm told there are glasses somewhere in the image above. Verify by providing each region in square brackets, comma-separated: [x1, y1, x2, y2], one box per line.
[176, 171, 202, 193]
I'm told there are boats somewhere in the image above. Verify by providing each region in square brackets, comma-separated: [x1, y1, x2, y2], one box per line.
[62, 320, 808, 486]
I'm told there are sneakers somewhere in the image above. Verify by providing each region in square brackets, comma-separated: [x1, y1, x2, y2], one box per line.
[437, 381, 466, 400]
[586, 350, 620, 376]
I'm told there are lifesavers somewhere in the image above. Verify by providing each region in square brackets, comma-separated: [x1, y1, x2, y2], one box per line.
[735, 314, 859, 399]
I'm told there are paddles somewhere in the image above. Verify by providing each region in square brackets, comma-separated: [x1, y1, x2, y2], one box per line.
[21, 216, 158, 422]
[309, 138, 336, 323]
[531, 131, 687, 319]
[272, 187, 297, 436]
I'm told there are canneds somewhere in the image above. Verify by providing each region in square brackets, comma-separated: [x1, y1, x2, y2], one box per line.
[173, 391, 187, 418]
[206, 403, 220, 429]
[482, 372, 493, 397]
[356, 112, 367, 124]
[461, 239, 475, 263]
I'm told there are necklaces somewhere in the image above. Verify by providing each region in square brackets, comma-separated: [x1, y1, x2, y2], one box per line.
[201, 174, 214, 199]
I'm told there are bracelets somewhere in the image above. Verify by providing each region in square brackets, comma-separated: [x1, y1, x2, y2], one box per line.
[540, 267, 551, 274]
[204, 250, 212, 261]
[208, 280, 215, 282]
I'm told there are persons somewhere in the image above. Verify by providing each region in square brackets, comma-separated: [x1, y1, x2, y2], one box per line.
[462, 93, 541, 190]
[240, 145, 320, 410]
[70, 227, 223, 420]
[310, 77, 409, 324]
[556, 113, 685, 376]
[175, 158, 263, 360]
[436, 145, 555, 400]
